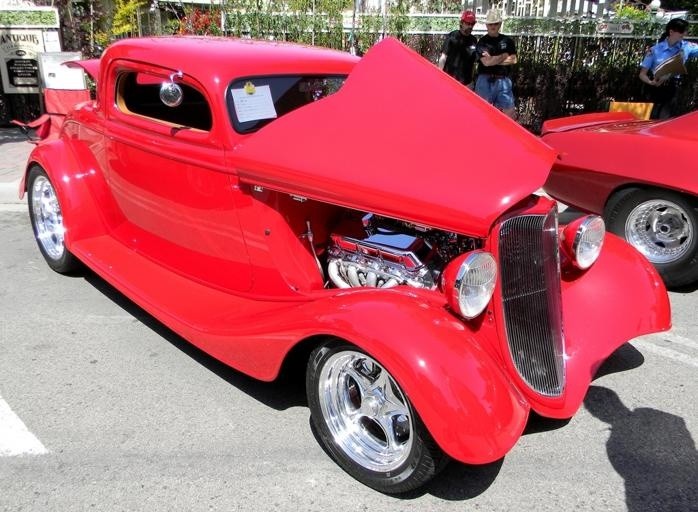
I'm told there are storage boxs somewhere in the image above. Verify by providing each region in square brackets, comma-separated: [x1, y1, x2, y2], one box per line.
[37, 53, 92, 140]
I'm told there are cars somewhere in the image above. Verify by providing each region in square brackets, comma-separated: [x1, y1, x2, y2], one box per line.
[7, 35, 671, 500]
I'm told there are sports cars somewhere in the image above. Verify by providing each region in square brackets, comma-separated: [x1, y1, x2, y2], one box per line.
[530, 108, 698, 293]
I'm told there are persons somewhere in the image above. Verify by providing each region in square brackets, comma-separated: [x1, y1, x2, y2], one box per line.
[638, 18, 698, 119]
[438, 10, 518, 121]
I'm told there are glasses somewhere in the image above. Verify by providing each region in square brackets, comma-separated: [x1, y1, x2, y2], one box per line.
[673, 28, 687, 33]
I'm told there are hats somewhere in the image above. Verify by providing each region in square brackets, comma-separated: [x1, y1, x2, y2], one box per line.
[478, 9, 509, 25]
[461, 11, 477, 23]
